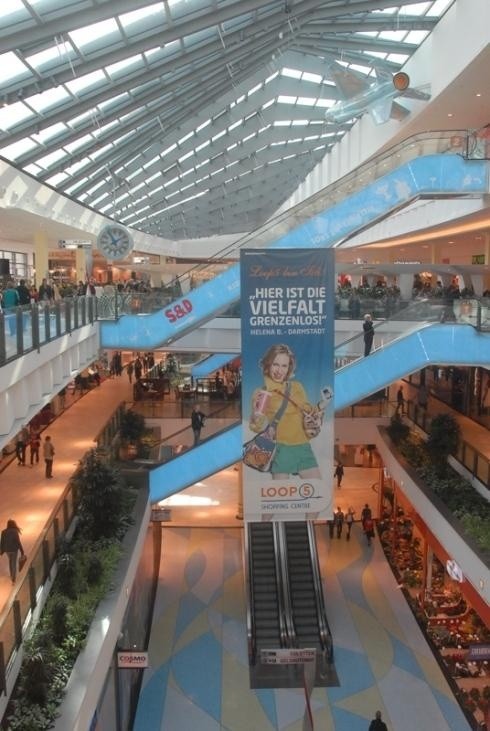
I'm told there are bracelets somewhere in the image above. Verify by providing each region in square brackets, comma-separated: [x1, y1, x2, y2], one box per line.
[316, 400, 325, 413]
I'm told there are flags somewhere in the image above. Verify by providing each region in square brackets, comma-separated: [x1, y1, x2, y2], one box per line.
[238, 248, 336, 524]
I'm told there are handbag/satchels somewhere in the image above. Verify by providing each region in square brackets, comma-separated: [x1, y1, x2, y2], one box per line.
[302, 408, 323, 439]
[243, 427, 276, 473]
[19, 555, 27, 572]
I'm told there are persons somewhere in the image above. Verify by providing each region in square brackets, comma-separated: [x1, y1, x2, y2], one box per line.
[412, 385, 433, 411]
[335, 463, 345, 488]
[43, 436, 55, 480]
[336, 506, 344, 540]
[326, 513, 338, 540]
[29, 425, 42, 467]
[345, 508, 356, 542]
[363, 516, 375, 546]
[1, 270, 174, 340]
[0, 518, 29, 588]
[367, 708, 389, 730]
[190, 403, 206, 448]
[213, 366, 240, 405]
[360, 503, 372, 531]
[71, 351, 182, 399]
[12, 428, 29, 468]
[337, 275, 489, 329]
[361, 312, 376, 359]
[394, 385, 407, 415]
[247, 342, 333, 523]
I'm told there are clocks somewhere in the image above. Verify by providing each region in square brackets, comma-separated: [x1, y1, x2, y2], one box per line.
[95, 225, 134, 262]
